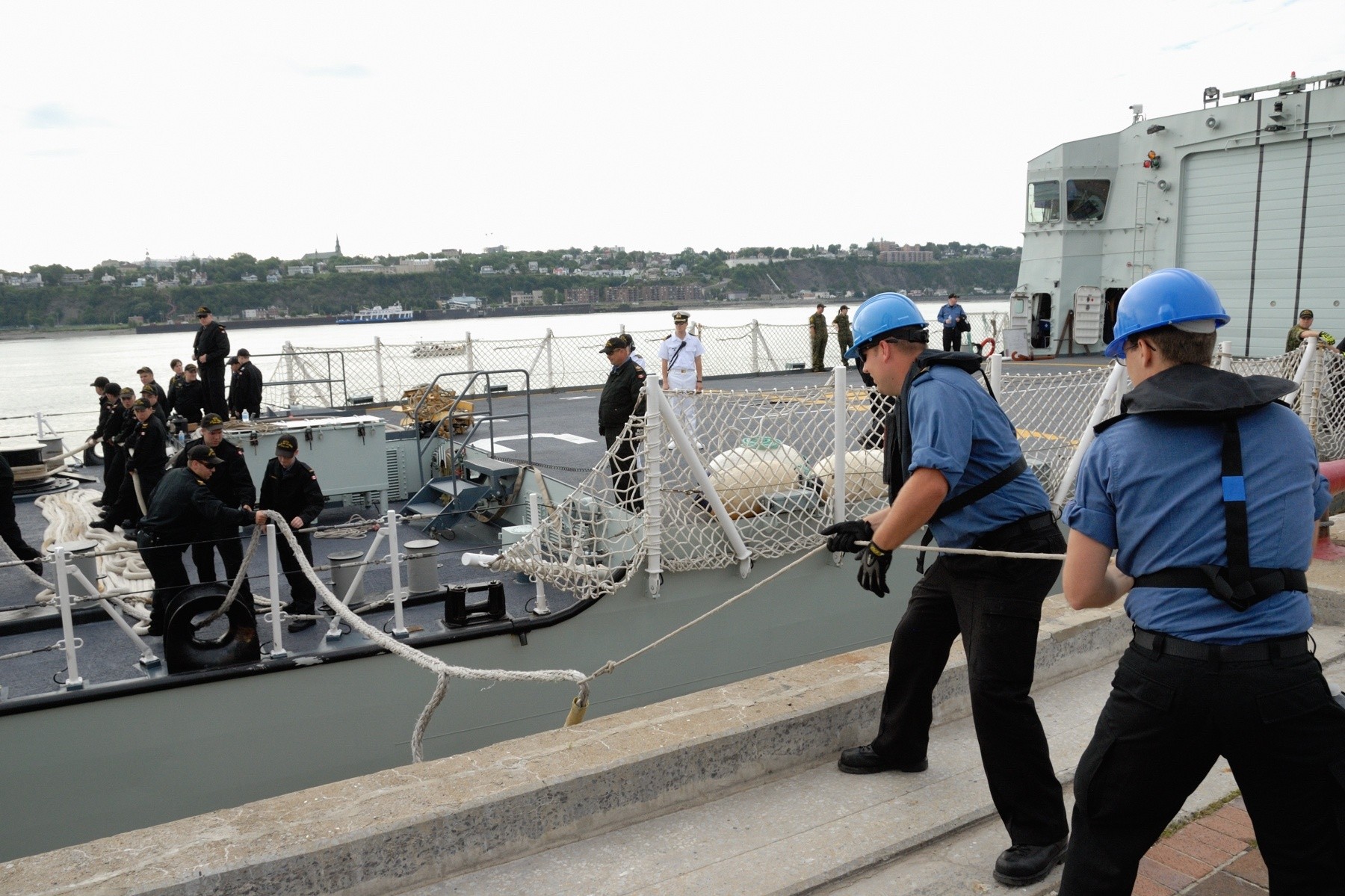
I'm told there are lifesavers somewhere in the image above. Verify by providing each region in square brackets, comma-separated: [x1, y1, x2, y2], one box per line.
[980, 338, 997, 358]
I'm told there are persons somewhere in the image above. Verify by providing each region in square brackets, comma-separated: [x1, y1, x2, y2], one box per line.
[658, 310, 706, 450]
[832, 305, 854, 370]
[855, 357, 896, 449]
[0, 454, 43, 576]
[820, 292, 1070, 887]
[1056, 269, 1345, 896]
[937, 293, 967, 352]
[136, 413, 326, 636]
[598, 333, 646, 513]
[809, 304, 829, 372]
[1282, 309, 1345, 432]
[85, 307, 262, 541]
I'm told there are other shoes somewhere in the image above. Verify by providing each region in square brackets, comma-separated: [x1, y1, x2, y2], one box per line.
[88, 500, 139, 542]
[35, 550, 44, 576]
[694, 437, 705, 449]
[283, 602, 301, 614]
[287, 617, 317, 633]
[668, 437, 676, 450]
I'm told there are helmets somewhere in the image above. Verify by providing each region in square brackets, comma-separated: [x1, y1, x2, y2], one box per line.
[1104, 267, 1231, 358]
[844, 292, 929, 358]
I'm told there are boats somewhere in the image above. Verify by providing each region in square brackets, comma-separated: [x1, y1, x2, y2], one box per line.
[409, 344, 466, 358]
[336, 300, 414, 325]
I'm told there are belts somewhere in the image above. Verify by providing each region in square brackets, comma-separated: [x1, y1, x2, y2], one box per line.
[670, 369, 694, 373]
[977, 514, 1053, 550]
[1132, 627, 1309, 660]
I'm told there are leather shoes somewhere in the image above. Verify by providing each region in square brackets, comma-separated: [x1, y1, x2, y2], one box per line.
[837, 743, 929, 773]
[993, 833, 1069, 886]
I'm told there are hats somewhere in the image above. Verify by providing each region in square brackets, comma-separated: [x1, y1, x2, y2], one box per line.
[185, 364, 199, 370]
[1300, 309, 1313, 318]
[817, 304, 826, 308]
[840, 305, 850, 310]
[948, 293, 960, 299]
[201, 413, 226, 432]
[620, 334, 633, 343]
[186, 444, 225, 464]
[226, 349, 253, 365]
[90, 367, 159, 410]
[197, 306, 212, 316]
[671, 310, 691, 321]
[598, 337, 626, 353]
[275, 434, 298, 458]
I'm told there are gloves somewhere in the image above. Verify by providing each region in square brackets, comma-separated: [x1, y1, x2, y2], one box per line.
[1319, 331, 1336, 346]
[855, 540, 893, 598]
[820, 519, 876, 554]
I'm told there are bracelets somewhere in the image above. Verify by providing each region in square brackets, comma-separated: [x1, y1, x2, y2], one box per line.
[697, 381, 702, 383]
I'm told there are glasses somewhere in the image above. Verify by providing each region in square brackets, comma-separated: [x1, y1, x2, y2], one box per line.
[857, 339, 898, 363]
[1113, 342, 1156, 367]
[196, 460, 215, 470]
[675, 321, 687, 325]
[605, 349, 620, 355]
